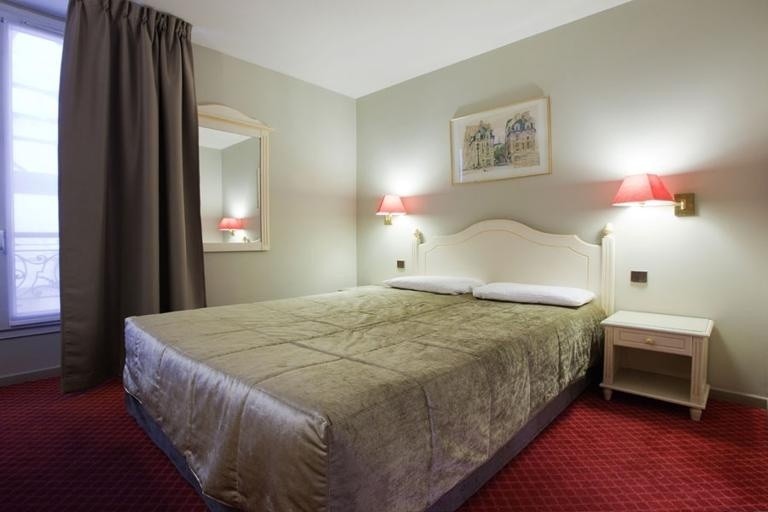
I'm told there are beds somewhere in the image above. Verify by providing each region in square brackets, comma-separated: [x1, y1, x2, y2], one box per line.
[116, 215, 620, 512]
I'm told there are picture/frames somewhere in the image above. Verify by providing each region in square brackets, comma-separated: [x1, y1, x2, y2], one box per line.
[445, 93, 554, 188]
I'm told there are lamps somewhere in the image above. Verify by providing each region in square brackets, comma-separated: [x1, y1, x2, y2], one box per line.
[609, 170, 698, 219]
[216, 214, 244, 242]
[374, 191, 406, 227]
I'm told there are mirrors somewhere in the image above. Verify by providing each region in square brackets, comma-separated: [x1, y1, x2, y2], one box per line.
[196, 100, 274, 254]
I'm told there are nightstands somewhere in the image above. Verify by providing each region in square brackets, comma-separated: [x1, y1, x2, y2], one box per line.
[593, 304, 714, 423]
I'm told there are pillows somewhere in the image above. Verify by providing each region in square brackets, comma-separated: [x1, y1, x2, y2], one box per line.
[378, 271, 483, 296]
[470, 279, 597, 309]
